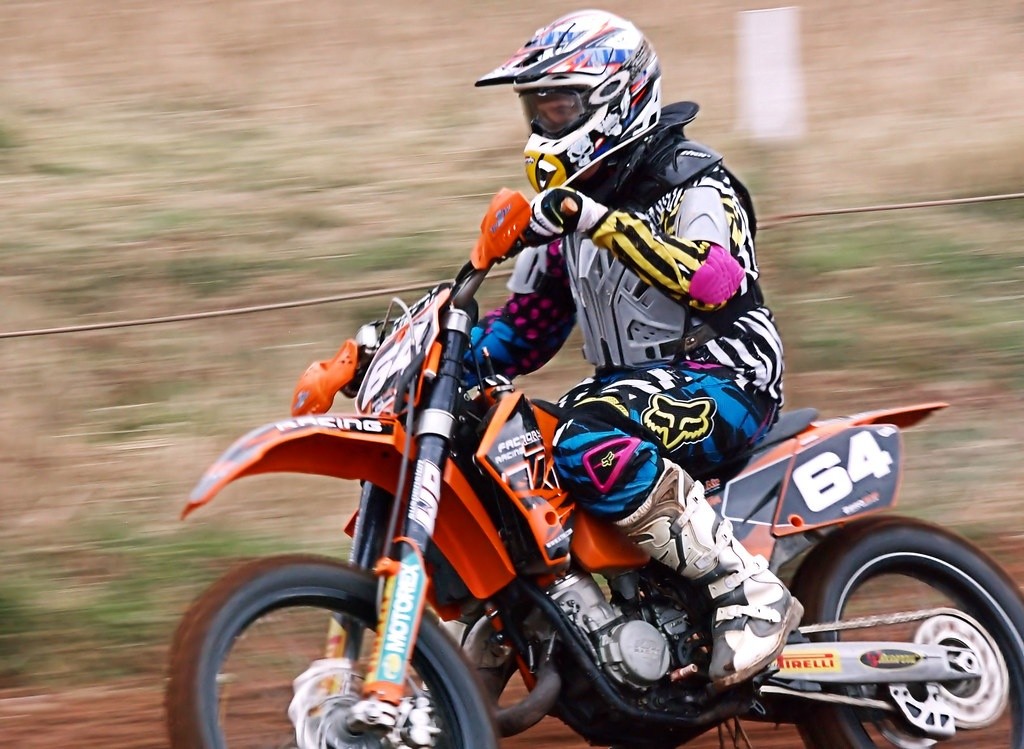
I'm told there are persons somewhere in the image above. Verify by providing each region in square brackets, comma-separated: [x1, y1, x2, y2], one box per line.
[338, 9, 805, 691]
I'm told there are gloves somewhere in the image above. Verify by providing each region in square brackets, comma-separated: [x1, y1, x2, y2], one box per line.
[527, 186, 606, 238]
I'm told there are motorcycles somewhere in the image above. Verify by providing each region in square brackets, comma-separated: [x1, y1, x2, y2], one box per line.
[162, 185, 1024, 749]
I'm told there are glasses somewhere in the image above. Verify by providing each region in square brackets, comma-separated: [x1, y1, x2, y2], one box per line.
[519, 35, 656, 140]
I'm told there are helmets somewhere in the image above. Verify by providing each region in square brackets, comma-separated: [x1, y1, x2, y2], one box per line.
[474, 8, 663, 194]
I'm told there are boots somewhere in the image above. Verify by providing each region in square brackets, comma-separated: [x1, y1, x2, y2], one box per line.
[614, 456, 804, 690]
